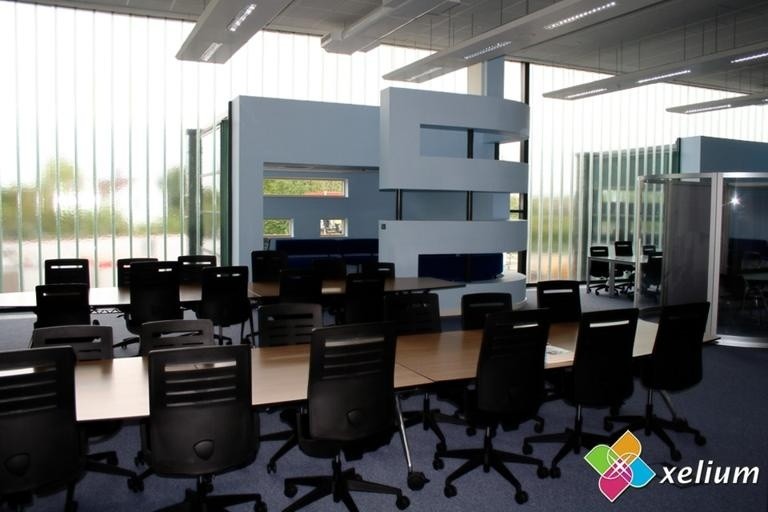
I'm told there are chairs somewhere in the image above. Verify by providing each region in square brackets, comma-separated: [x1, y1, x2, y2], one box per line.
[717, 270, 768, 332]
[585, 239, 664, 300]
